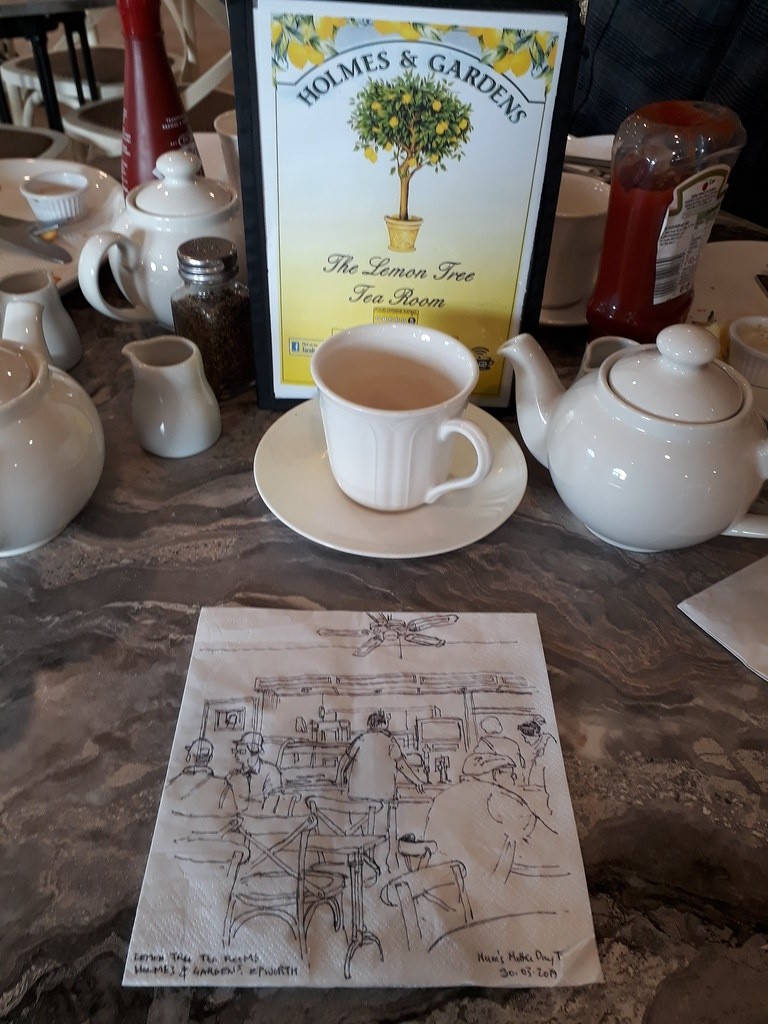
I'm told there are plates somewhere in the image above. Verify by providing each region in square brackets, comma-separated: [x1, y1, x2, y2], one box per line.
[565, 134, 615, 173]
[0, 158, 124, 292]
[253, 400, 528, 559]
[538, 296, 591, 326]
[684, 239, 768, 328]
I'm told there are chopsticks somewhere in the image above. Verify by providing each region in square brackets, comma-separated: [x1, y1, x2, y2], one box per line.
[565, 156, 612, 167]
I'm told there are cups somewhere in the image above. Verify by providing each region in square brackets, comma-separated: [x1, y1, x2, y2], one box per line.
[311, 323, 496, 513]
[213, 108, 240, 189]
[728, 316, 767, 417]
[568, 336, 641, 391]
[541, 173, 610, 308]
[20, 171, 90, 227]
[121, 336, 223, 458]
[0, 269, 83, 374]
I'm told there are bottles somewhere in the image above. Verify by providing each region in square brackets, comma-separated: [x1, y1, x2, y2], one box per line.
[584, 101, 745, 342]
[115, 0, 207, 204]
[172, 238, 258, 400]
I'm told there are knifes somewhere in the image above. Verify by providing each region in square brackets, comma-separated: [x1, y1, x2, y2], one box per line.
[0, 226, 71, 265]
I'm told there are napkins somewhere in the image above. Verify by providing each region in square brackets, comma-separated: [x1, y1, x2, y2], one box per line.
[565, 134, 615, 176]
[676, 555, 767, 684]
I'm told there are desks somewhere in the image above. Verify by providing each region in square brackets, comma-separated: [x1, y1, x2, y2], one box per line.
[0, 282, 767, 1024]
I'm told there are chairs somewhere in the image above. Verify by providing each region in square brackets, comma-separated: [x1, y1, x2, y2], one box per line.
[0, 0, 235, 180]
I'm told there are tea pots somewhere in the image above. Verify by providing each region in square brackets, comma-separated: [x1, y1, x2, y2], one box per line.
[497, 322, 768, 554]
[0, 301, 105, 557]
[77, 151, 247, 328]
